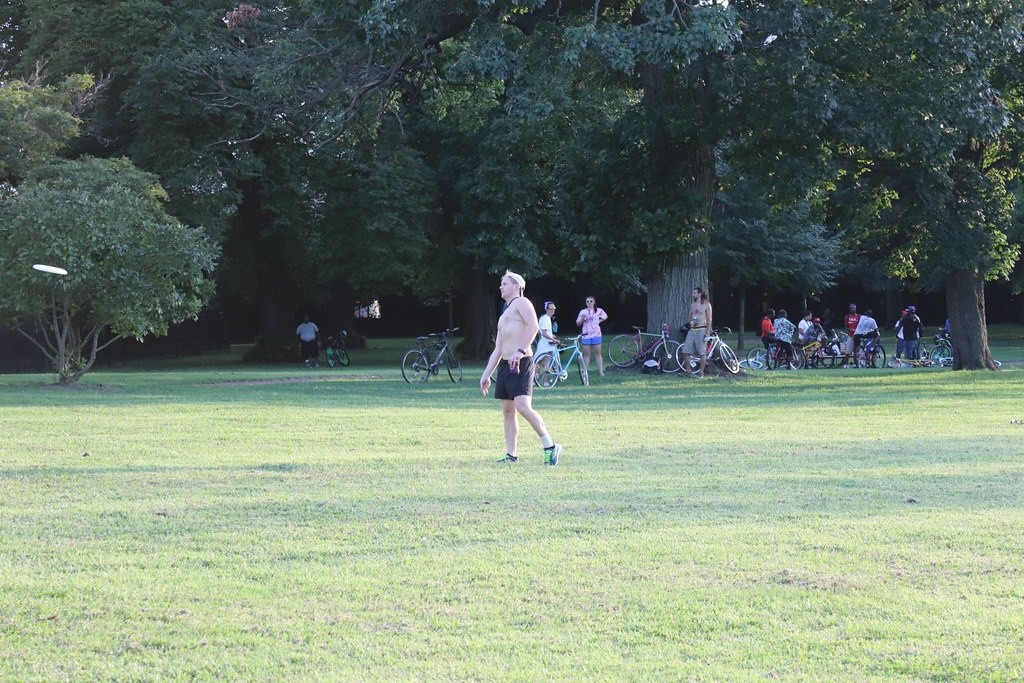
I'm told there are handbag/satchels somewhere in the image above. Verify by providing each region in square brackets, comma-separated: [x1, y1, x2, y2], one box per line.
[642, 357, 662, 375]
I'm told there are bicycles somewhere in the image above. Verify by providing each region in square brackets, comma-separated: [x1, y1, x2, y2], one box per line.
[887, 353, 935, 369]
[608, 321, 686, 374]
[325, 329, 349, 368]
[853, 329, 886, 369]
[675, 327, 740, 374]
[533, 333, 589, 389]
[401, 327, 463, 385]
[939, 357, 1002, 368]
[920, 342, 932, 360]
[798, 329, 849, 369]
[551, 355, 561, 381]
[746, 341, 803, 371]
[738, 352, 774, 370]
[766, 337, 807, 371]
[929, 327, 952, 366]
[487, 354, 498, 382]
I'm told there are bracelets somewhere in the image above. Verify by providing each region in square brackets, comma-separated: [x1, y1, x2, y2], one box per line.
[518, 349, 525, 355]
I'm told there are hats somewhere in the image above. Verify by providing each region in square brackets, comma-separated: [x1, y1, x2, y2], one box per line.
[906, 306, 917, 312]
[849, 304, 856, 309]
[813, 318, 820, 323]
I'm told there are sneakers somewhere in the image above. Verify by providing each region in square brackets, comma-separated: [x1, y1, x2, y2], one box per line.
[543, 443, 562, 466]
[496, 453, 519, 464]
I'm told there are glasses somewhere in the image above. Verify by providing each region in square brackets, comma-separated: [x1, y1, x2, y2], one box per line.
[585, 300, 593, 303]
[547, 306, 556, 309]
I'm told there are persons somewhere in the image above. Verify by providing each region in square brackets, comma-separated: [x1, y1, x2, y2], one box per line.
[534, 301, 566, 386]
[682, 287, 712, 379]
[297, 312, 320, 368]
[576, 296, 608, 376]
[480, 269, 562, 466]
[762, 304, 951, 370]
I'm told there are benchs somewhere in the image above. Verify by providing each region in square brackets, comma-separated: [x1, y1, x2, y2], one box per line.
[803, 341, 857, 367]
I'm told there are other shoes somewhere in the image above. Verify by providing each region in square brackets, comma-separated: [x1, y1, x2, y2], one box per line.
[697, 373, 704, 379]
[313, 363, 320, 369]
[676, 372, 692, 378]
[304, 363, 312, 369]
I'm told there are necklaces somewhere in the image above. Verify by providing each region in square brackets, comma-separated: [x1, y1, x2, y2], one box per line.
[503, 297, 519, 312]
